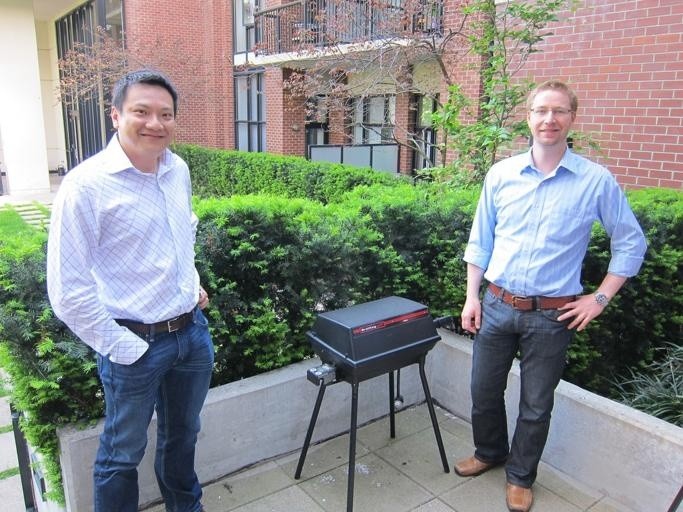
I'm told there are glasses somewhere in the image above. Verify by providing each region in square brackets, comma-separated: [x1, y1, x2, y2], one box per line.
[529, 105, 573, 116]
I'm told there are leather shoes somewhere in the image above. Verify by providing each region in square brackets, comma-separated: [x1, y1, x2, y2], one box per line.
[505, 482, 533, 512]
[454, 458, 505, 476]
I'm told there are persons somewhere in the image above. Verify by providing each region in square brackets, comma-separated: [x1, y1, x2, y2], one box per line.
[46, 69, 215, 512]
[452, 79, 648, 512]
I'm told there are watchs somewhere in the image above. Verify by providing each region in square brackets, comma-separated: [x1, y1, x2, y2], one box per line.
[593, 290, 609, 307]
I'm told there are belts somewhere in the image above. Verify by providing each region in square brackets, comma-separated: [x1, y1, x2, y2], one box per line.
[116, 313, 192, 335]
[489, 284, 574, 312]
